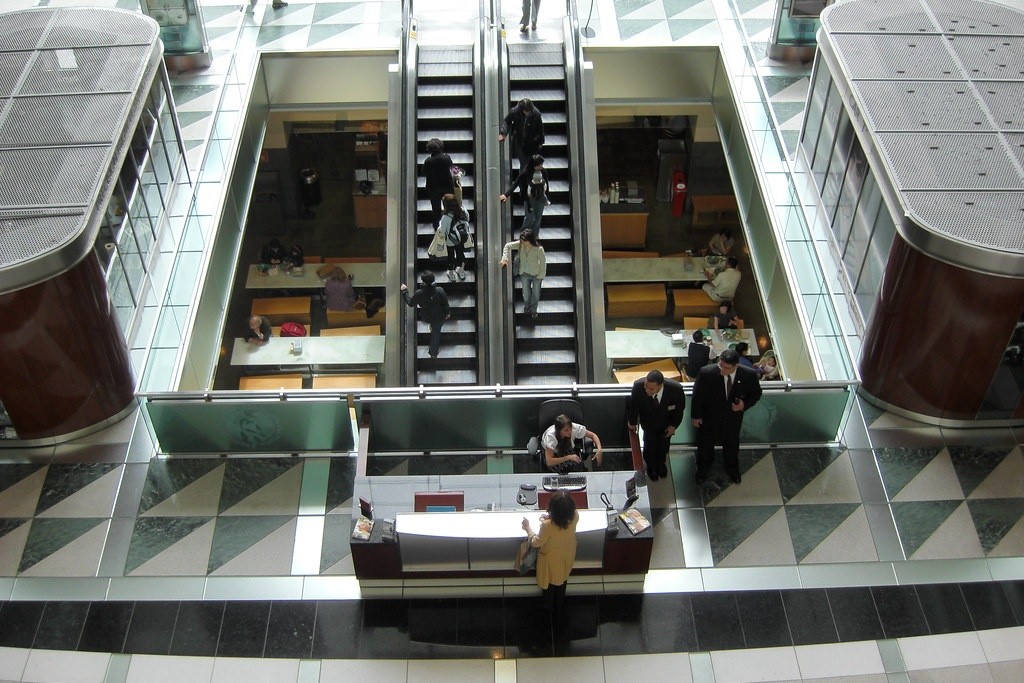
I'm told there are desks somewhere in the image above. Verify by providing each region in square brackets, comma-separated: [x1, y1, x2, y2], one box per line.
[230, 333, 391, 369]
[606, 328, 760, 358]
[603, 257, 730, 284]
[243, 259, 388, 290]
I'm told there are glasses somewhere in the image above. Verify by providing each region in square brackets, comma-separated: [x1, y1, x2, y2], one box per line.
[520, 236, 527, 242]
[718, 361, 734, 372]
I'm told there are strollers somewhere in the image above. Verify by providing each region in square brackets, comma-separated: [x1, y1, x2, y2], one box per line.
[753, 350, 779, 381]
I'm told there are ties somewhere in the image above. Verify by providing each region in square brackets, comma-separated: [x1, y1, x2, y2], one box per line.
[654, 394, 658, 404]
[727, 375, 732, 400]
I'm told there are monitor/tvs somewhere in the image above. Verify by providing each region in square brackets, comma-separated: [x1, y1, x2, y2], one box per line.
[413, 492, 465, 512]
[538, 491, 588, 510]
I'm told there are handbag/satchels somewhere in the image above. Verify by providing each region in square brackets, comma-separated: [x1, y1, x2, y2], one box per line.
[428, 228, 445, 255]
[513, 242, 522, 276]
[454, 180, 462, 206]
[514, 532, 538, 575]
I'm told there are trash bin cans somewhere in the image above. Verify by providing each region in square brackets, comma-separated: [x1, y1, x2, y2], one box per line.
[298, 169, 321, 207]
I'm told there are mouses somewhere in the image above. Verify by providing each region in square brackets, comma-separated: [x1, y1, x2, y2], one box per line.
[519, 494, 527, 502]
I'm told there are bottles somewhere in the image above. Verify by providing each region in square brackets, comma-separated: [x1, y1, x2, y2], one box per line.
[615, 182, 620, 203]
[609, 183, 615, 203]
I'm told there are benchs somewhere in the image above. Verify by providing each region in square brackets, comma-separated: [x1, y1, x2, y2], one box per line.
[690, 195, 739, 225]
[239, 251, 387, 391]
[604, 243, 764, 386]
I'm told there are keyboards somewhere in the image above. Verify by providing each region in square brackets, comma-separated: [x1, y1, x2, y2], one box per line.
[542, 476, 573, 490]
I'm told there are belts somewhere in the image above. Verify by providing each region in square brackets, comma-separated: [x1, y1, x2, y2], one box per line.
[717, 294, 729, 298]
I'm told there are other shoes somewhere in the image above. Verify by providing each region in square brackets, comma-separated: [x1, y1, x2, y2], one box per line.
[521, 25, 528, 31]
[647, 462, 658, 481]
[696, 466, 711, 486]
[447, 269, 456, 281]
[532, 24, 536, 30]
[658, 463, 667, 478]
[455, 266, 466, 279]
[729, 470, 741, 483]
[524, 303, 538, 318]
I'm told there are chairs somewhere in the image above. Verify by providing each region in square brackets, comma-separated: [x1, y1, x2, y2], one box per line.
[538, 399, 588, 467]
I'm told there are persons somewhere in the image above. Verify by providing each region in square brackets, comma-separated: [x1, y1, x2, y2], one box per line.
[439, 194, 469, 281]
[324, 267, 355, 311]
[760, 357, 776, 373]
[500, 228, 546, 323]
[628, 370, 685, 482]
[501, 154, 551, 237]
[244, 315, 272, 346]
[401, 271, 451, 358]
[703, 257, 741, 302]
[707, 227, 734, 256]
[423, 138, 454, 227]
[261, 238, 304, 267]
[520, 0, 541, 32]
[735, 342, 766, 373]
[714, 300, 739, 335]
[691, 349, 762, 487]
[685, 331, 717, 378]
[522, 489, 578, 610]
[541, 414, 603, 472]
[499, 99, 546, 174]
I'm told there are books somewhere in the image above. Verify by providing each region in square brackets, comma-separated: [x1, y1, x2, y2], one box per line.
[619, 507, 652, 536]
[352, 518, 375, 541]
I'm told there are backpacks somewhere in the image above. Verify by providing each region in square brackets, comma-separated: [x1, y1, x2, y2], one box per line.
[280, 322, 306, 337]
[446, 206, 470, 246]
